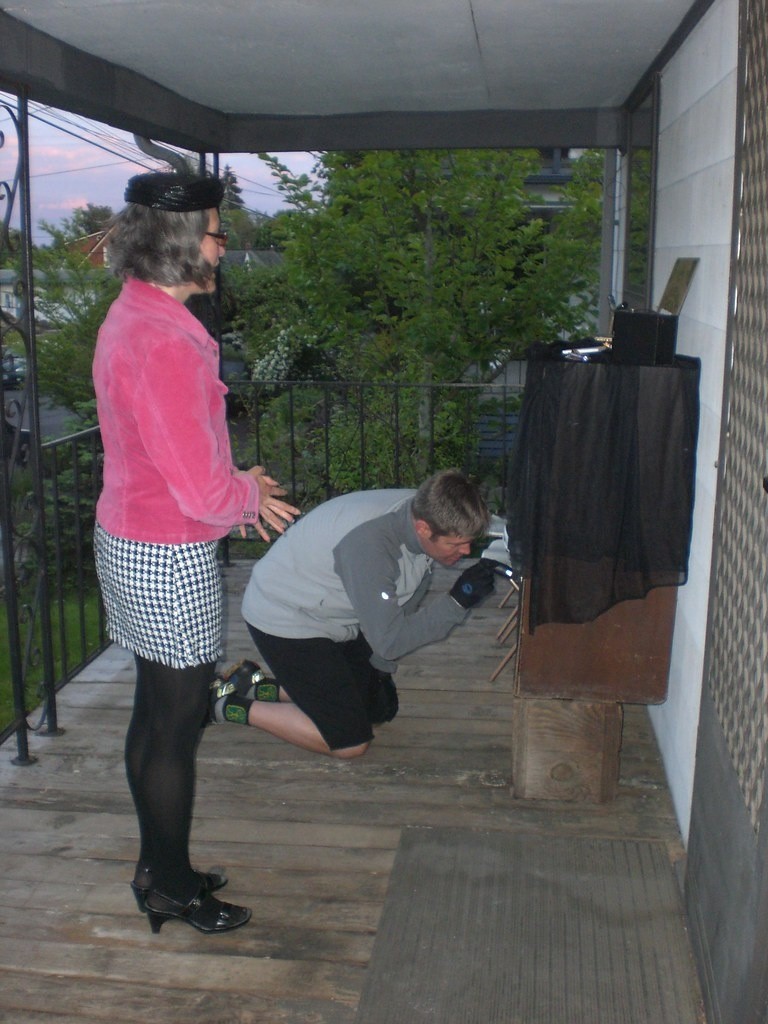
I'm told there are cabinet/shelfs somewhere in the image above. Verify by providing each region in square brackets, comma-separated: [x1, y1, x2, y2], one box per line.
[512, 353, 700, 707]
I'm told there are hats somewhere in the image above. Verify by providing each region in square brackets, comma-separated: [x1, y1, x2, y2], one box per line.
[125, 173, 224, 212]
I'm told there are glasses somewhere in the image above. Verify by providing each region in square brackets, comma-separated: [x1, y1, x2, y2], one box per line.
[204, 230, 227, 246]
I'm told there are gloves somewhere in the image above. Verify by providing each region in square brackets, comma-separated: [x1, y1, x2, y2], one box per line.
[450, 558, 499, 610]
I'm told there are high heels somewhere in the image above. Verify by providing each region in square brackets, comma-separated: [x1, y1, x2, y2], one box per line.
[143, 888, 251, 934]
[130, 868, 228, 913]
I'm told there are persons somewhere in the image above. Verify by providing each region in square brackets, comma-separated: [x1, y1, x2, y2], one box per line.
[205, 466, 500, 761]
[92, 172, 300, 936]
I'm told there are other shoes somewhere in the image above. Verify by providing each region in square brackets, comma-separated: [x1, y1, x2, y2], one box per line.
[205, 674, 236, 727]
[223, 658, 266, 698]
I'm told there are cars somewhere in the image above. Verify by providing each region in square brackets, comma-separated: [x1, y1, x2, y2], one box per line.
[3, 357, 26, 389]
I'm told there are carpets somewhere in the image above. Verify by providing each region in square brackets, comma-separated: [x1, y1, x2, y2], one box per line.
[353, 824, 702, 1024]
